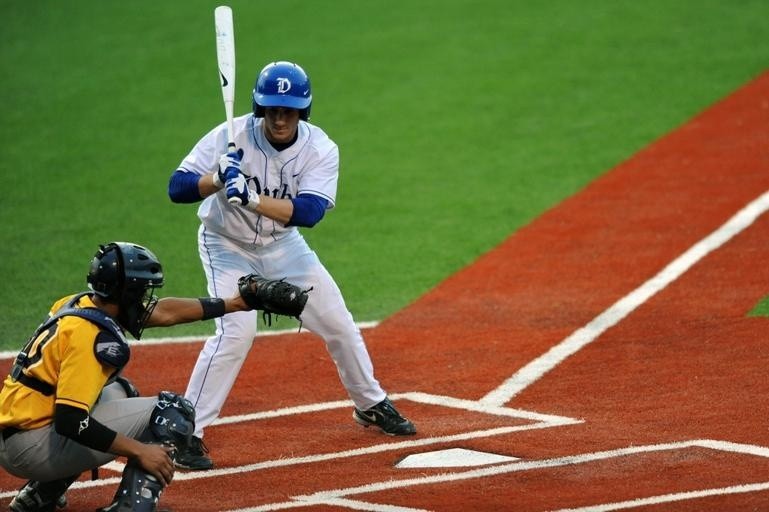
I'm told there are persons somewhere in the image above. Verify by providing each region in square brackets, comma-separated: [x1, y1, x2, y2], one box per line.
[166, 60, 418, 471]
[1, 239, 314, 511]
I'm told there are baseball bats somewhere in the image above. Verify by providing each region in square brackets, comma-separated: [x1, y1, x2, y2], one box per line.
[214, 5, 245, 210]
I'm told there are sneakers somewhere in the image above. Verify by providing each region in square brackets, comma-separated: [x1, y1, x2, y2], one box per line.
[6, 484, 67, 511]
[172, 433, 216, 472]
[349, 397, 420, 438]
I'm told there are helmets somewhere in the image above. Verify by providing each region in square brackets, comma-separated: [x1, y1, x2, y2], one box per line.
[85, 240, 166, 305]
[250, 57, 312, 125]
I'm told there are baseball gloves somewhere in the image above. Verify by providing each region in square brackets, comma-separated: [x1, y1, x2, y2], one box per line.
[238, 274, 308, 315]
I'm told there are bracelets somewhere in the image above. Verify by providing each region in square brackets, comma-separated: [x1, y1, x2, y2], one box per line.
[244, 188, 260, 212]
[213, 171, 225, 189]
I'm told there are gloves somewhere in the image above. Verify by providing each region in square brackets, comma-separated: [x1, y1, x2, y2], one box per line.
[213, 149, 260, 212]
